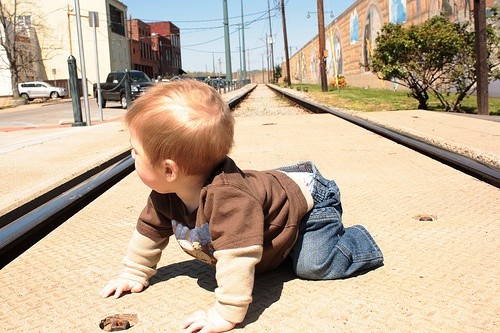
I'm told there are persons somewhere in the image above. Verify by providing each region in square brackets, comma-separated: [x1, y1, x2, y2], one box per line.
[102, 78, 384, 331]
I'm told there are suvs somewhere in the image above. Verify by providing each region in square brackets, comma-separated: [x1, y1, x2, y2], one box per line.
[17, 82, 67, 102]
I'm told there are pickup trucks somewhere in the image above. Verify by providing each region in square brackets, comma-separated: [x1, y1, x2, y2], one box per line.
[93, 70, 156, 109]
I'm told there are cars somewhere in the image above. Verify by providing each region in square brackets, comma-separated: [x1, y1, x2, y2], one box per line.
[199, 76, 237, 89]
